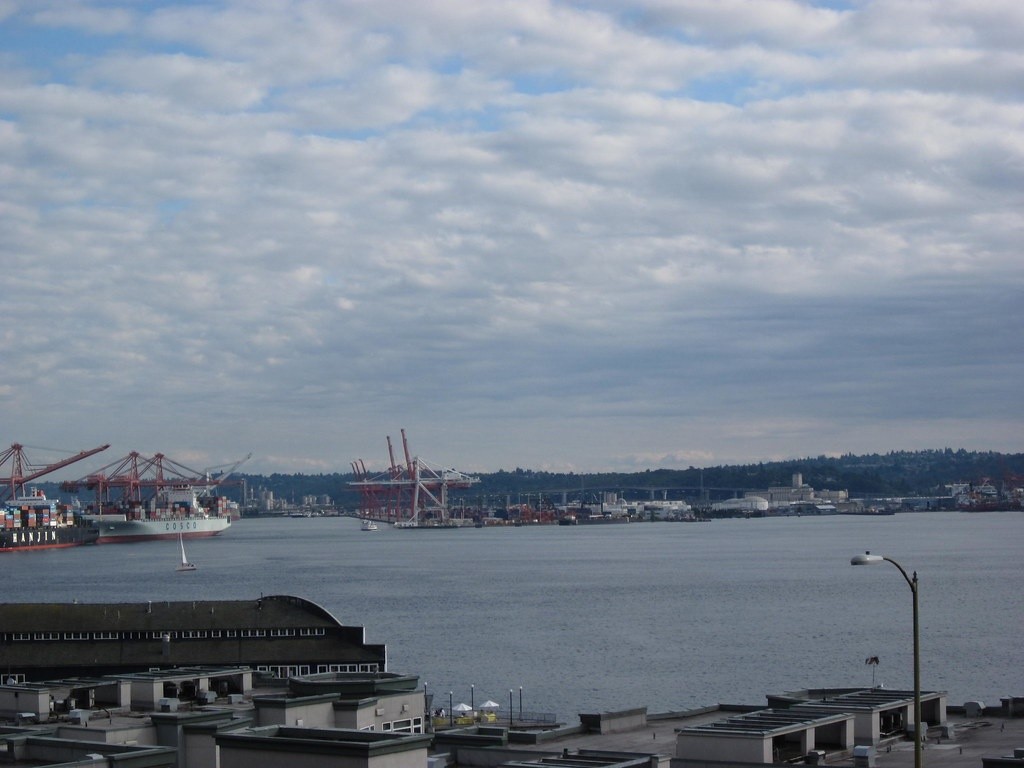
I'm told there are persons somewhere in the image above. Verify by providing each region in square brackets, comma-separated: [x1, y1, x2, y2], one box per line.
[432, 707, 495, 719]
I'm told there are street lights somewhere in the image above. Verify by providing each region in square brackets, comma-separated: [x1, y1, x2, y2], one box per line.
[470, 684, 474, 710]
[519, 686, 522, 720]
[424, 681, 428, 714]
[509, 689, 513, 725]
[850, 550, 922, 768]
[448, 691, 453, 726]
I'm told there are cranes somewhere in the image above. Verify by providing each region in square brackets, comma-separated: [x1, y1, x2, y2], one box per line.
[0, 439, 112, 519]
[61, 450, 254, 515]
[343, 427, 482, 525]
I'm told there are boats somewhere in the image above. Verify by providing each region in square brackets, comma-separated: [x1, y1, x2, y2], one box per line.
[70, 494, 231, 545]
[0, 508, 99, 551]
[5, 485, 58, 508]
[361, 520, 377, 531]
